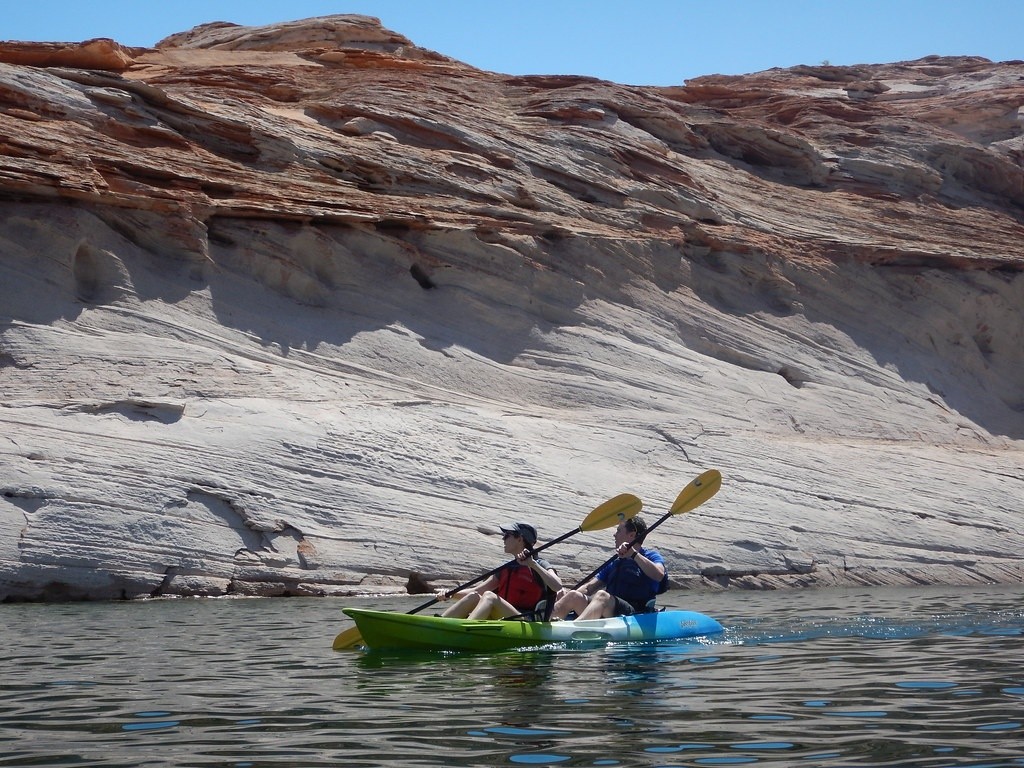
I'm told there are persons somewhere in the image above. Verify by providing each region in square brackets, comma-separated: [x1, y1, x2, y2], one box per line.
[551, 517, 669, 620]
[437, 523, 562, 618]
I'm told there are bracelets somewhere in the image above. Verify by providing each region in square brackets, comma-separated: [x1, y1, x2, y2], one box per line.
[631, 551, 638, 559]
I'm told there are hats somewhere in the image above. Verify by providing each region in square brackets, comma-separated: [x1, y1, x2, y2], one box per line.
[500, 524, 537, 546]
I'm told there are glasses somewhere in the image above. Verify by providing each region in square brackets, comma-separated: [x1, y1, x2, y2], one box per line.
[504, 531, 516, 537]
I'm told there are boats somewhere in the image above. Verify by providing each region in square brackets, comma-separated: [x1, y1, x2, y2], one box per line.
[341, 610, 727, 650]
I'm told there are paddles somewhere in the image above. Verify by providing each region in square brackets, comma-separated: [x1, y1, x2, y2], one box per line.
[570, 468, 723, 588]
[334, 492, 642, 651]
